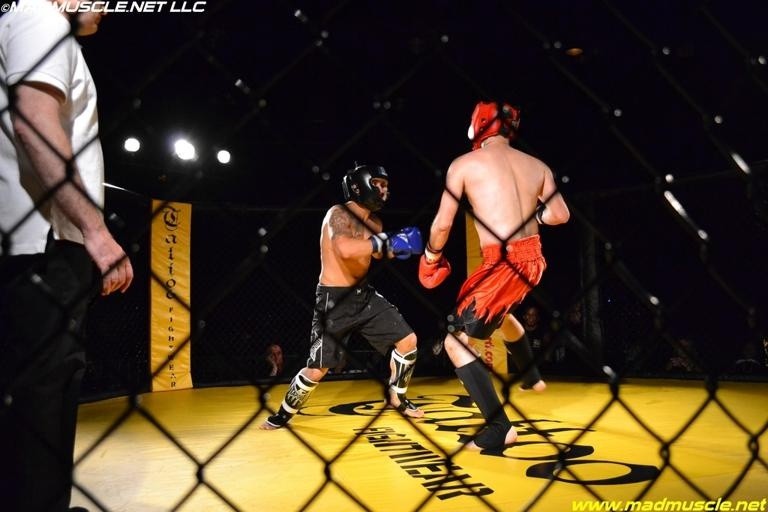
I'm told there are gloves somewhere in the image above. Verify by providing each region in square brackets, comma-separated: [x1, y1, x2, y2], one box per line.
[417, 244, 452, 289]
[370, 225, 423, 259]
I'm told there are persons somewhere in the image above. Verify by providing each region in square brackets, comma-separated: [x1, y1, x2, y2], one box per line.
[264, 160, 427, 429]
[252, 344, 285, 378]
[418, 100, 574, 449]
[0, 0, 135, 512]
[518, 299, 768, 381]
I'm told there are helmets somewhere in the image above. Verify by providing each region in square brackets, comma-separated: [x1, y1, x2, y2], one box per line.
[467, 100, 522, 150]
[341, 163, 389, 212]
